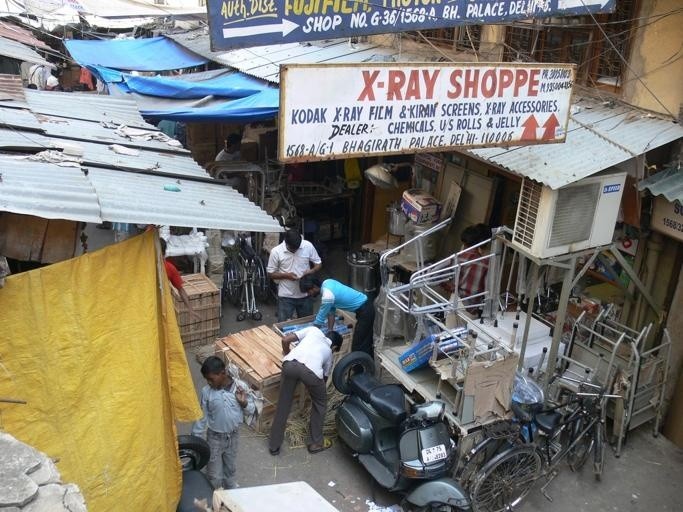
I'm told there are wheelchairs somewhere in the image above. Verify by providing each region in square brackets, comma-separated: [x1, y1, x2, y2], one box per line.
[225, 245, 268, 321]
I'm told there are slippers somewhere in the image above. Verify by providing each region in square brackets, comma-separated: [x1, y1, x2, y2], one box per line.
[270, 448, 280, 455]
[309, 440, 332, 453]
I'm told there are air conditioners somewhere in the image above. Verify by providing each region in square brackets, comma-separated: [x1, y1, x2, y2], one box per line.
[512, 167, 628, 259]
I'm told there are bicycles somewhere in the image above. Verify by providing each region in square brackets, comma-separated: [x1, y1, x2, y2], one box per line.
[458, 376, 627, 512]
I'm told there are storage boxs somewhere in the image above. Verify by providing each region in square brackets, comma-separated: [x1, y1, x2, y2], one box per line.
[273, 307, 357, 387]
[169, 274, 222, 349]
[401, 189, 439, 224]
[214, 325, 300, 435]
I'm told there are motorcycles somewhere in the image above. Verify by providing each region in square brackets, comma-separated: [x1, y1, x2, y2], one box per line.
[330, 351, 472, 512]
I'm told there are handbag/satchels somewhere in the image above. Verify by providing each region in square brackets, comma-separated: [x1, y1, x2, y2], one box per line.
[228, 364, 268, 427]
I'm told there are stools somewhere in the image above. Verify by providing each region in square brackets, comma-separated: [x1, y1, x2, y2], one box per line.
[429, 331, 493, 385]
[437, 376, 463, 416]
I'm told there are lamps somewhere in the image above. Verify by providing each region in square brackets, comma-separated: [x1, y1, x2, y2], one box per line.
[366, 160, 414, 189]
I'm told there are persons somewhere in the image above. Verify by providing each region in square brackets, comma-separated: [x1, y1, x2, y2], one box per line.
[175, 119, 185, 145]
[444, 223, 492, 315]
[265, 231, 322, 323]
[457, 226, 477, 256]
[160, 236, 201, 317]
[288, 164, 304, 182]
[298, 274, 374, 376]
[157, 117, 175, 138]
[20, 60, 89, 92]
[269, 324, 344, 456]
[214, 134, 241, 161]
[193, 357, 254, 493]
